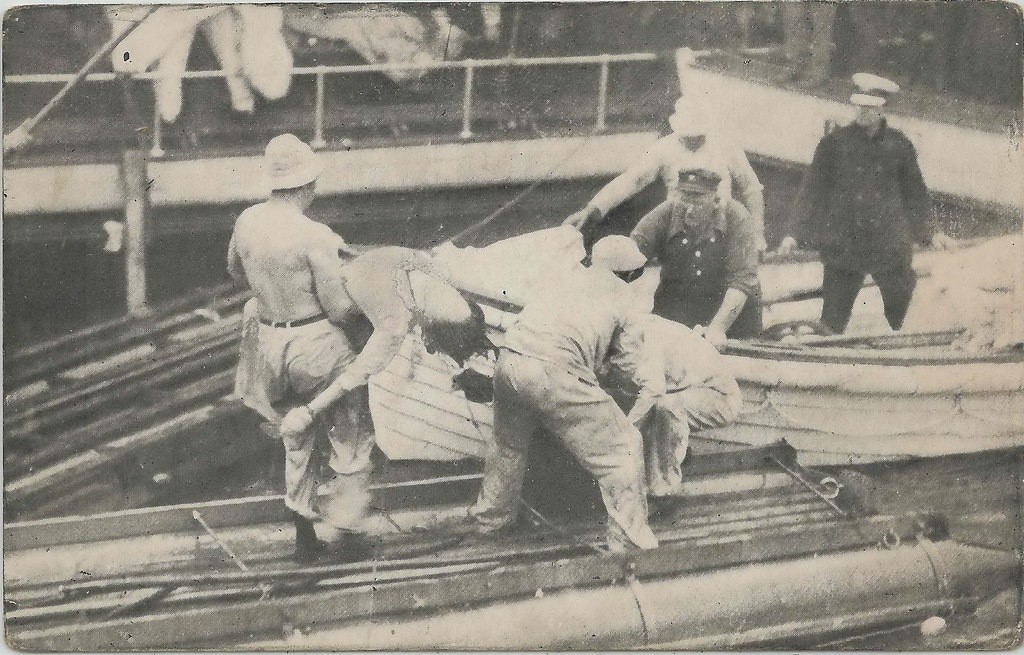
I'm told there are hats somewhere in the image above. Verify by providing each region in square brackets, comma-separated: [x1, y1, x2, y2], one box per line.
[848, 71, 899, 108]
[668, 94, 714, 138]
[589, 234, 648, 272]
[262, 132, 324, 192]
[675, 166, 722, 194]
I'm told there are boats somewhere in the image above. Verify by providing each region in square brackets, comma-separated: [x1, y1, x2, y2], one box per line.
[1, 219, 1024, 653]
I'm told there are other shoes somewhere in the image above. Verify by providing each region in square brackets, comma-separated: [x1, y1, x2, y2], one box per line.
[291, 539, 331, 564]
[334, 540, 373, 564]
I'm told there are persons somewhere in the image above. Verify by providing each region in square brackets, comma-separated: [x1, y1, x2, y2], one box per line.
[562, 112, 767, 350]
[226, 131, 488, 564]
[777, 72, 956, 334]
[611, 314, 743, 499]
[469, 235, 658, 554]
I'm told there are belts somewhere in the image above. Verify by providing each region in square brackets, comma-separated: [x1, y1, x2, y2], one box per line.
[258, 311, 328, 328]
